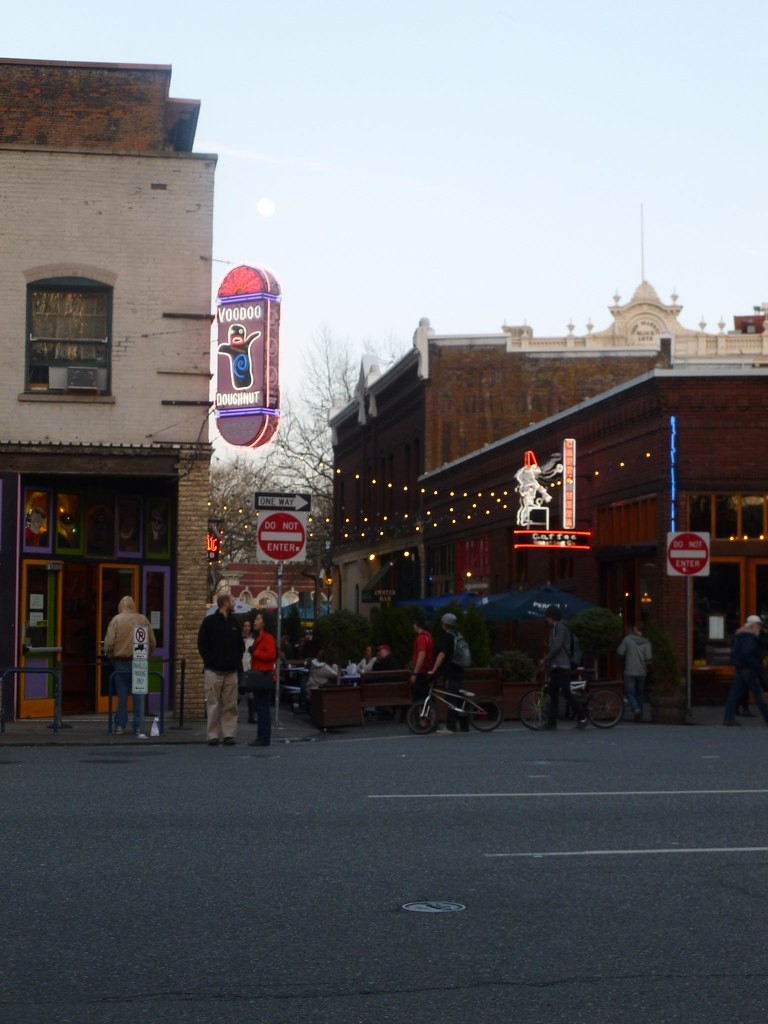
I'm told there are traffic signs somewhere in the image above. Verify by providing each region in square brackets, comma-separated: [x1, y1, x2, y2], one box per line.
[253, 491, 313, 512]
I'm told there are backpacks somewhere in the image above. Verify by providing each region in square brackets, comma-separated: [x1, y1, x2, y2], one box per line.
[570, 632, 584, 669]
[448, 630, 472, 668]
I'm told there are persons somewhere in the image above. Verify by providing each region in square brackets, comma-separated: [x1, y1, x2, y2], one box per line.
[371, 643, 399, 682]
[248, 611, 276, 746]
[104, 596, 156, 735]
[357, 644, 376, 721]
[305, 647, 338, 700]
[427, 613, 469, 734]
[237, 619, 258, 724]
[279, 635, 316, 708]
[723, 615, 768, 726]
[537, 603, 588, 730]
[617, 620, 652, 721]
[197, 594, 246, 746]
[410, 616, 438, 732]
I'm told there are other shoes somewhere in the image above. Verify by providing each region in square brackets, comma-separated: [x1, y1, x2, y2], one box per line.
[537, 721, 557, 729]
[208, 739, 219, 745]
[736, 709, 758, 718]
[224, 737, 236, 745]
[634, 709, 643, 721]
[113, 727, 124, 735]
[436, 727, 455, 734]
[724, 720, 740, 726]
[248, 740, 271, 746]
[134, 727, 141, 735]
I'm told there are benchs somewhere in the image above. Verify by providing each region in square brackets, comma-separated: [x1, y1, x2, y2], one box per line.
[278, 683, 303, 695]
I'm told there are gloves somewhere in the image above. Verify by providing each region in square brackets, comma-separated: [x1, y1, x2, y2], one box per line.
[248, 645, 255, 653]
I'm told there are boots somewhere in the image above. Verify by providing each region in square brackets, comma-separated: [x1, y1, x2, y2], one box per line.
[248, 701, 257, 724]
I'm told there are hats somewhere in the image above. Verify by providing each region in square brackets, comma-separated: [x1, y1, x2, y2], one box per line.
[744, 615, 761, 628]
[375, 644, 391, 651]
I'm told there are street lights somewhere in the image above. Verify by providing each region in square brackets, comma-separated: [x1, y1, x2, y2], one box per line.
[325, 577, 331, 614]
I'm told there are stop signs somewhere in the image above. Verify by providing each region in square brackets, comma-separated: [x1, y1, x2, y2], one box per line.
[668, 533, 708, 574]
[259, 512, 305, 560]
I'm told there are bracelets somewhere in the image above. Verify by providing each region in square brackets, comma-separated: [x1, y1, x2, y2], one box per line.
[412, 668, 418, 676]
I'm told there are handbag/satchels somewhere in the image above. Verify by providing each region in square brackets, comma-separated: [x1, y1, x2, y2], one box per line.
[248, 670, 275, 689]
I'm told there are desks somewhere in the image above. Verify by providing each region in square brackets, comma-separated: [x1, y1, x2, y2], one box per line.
[300, 668, 310, 692]
[337, 674, 361, 686]
[284, 666, 306, 687]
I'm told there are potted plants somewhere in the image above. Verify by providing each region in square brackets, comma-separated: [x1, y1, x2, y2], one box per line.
[463, 600, 502, 699]
[571, 605, 624, 718]
[495, 650, 540, 719]
[308, 609, 372, 729]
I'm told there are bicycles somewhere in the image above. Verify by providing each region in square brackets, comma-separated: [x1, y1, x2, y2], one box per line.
[406, 674, 503, 735]
[518, 673, 626, 732]
